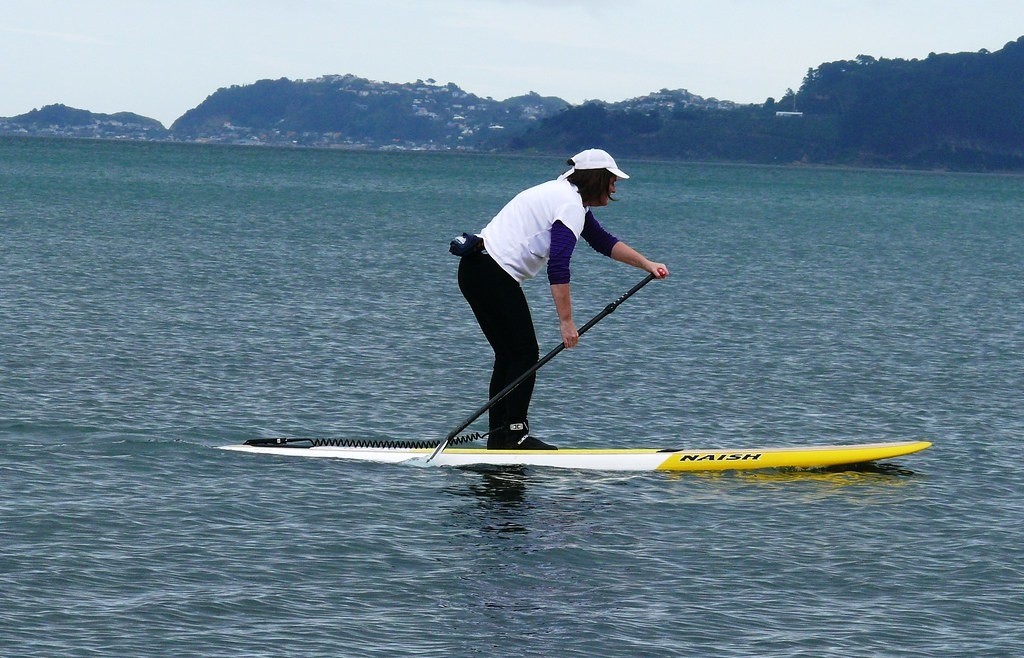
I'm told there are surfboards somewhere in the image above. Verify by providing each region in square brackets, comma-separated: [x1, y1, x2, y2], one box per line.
[215, 441, 933, 472]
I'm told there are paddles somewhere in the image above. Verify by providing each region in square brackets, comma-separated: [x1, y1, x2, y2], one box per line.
[425, 268, 667, 465]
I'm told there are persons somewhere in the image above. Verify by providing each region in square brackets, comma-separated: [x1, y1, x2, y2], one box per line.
[457, 148, 669, 450]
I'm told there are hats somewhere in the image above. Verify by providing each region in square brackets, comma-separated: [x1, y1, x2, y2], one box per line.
[558, 148, 630, 181]
